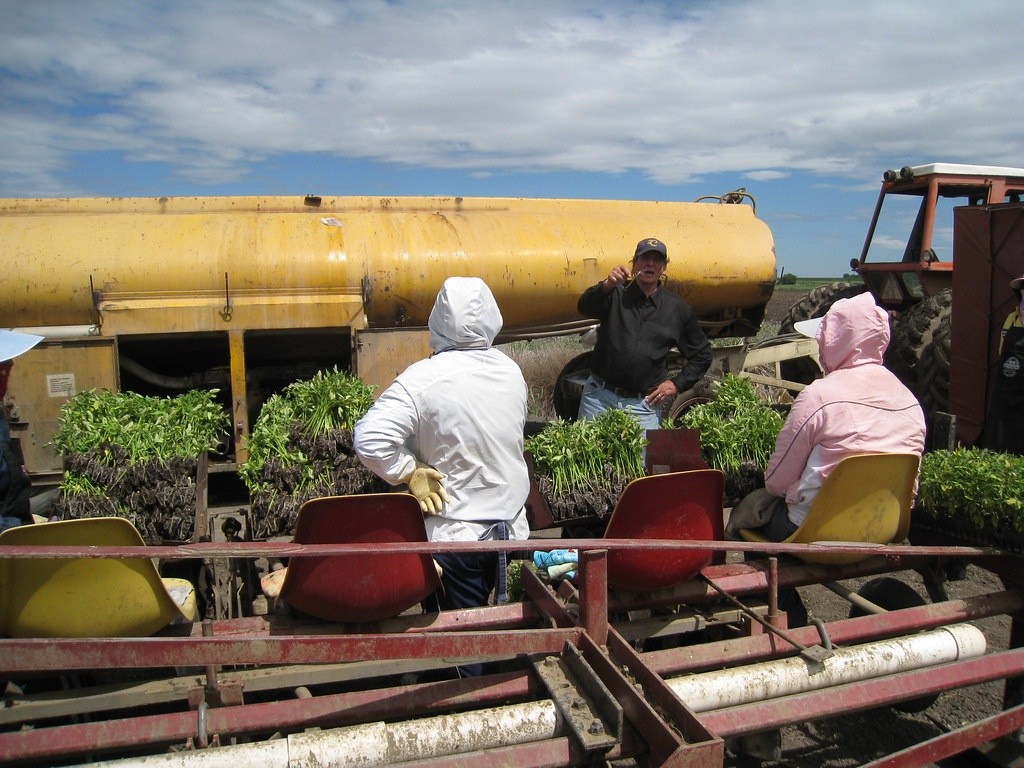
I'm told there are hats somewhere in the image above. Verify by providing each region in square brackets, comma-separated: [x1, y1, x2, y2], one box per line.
[794, 317, 823, 339]
[635, 238, 667, 259]
[1009, 274, 1024, 289]
[0, 329, 45, 363]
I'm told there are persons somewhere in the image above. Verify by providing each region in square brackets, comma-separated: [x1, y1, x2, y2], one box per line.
[732, 291, 927, 630]
[0, 330, 45, 531]
[353, 276, 530, 681]
[577, 238, 713, 467]
[974, 275, 1024, 456]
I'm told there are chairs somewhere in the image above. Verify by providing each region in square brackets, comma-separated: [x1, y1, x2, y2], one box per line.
[272, 493, 446, 623]
[0, 516, 197, 638]
[554, 469, 727, 591]
[738, 453, 919, 565]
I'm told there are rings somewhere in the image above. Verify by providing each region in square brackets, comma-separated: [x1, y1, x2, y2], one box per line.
[658, 394, 661, 397]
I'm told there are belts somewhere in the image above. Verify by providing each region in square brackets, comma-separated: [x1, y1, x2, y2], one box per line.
[590, 371, 647, 398]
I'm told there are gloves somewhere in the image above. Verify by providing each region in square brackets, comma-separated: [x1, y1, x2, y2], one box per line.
[403, 460, 449, 515]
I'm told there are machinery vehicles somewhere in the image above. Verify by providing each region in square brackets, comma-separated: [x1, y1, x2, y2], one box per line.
[775, 162, 1024, 453]
